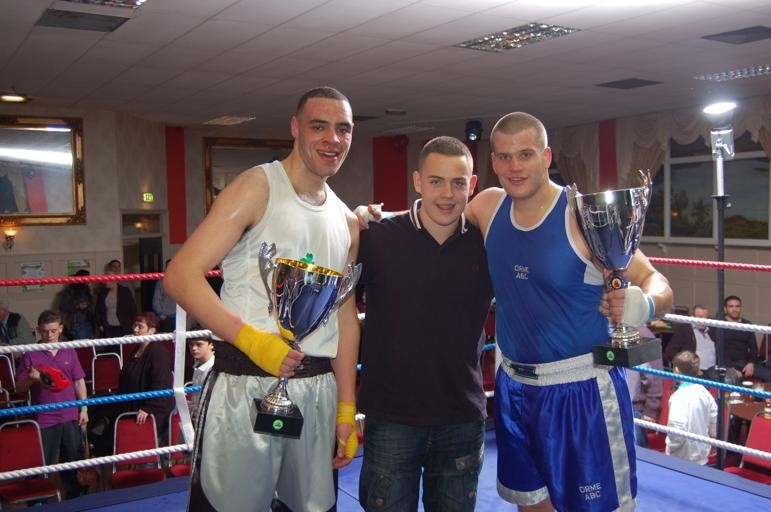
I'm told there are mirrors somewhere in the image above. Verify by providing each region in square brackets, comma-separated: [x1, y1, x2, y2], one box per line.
[203, 138, 295, 220]
[1, 115, 88, 228]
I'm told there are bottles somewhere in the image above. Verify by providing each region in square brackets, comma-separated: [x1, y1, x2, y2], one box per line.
[764, 398, 771, 419]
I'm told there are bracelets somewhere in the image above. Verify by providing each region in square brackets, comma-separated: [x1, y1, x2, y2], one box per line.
[80, 405, 88, 411]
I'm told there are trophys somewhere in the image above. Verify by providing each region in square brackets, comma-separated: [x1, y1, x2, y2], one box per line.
[562, 169, 653, 349]
[250, 240, 360, 440]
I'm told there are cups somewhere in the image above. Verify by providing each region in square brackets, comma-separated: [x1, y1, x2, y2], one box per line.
[742, 381, 754, 405]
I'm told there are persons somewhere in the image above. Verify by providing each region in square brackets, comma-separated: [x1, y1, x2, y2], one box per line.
[349, 135, 613, 512]
[177, 338, 216, 469]
[1, 258, 177, 366]
[349, 109, 673, 510]
[112, 312, 175, 471]
[161, 85, 361, 512]
[613, 294, 771, 468]
[15, 310, 91, 506]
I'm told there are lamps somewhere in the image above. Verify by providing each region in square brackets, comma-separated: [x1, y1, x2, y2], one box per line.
[4, 229, 17, 249]
[702, 100, 739, 469]
